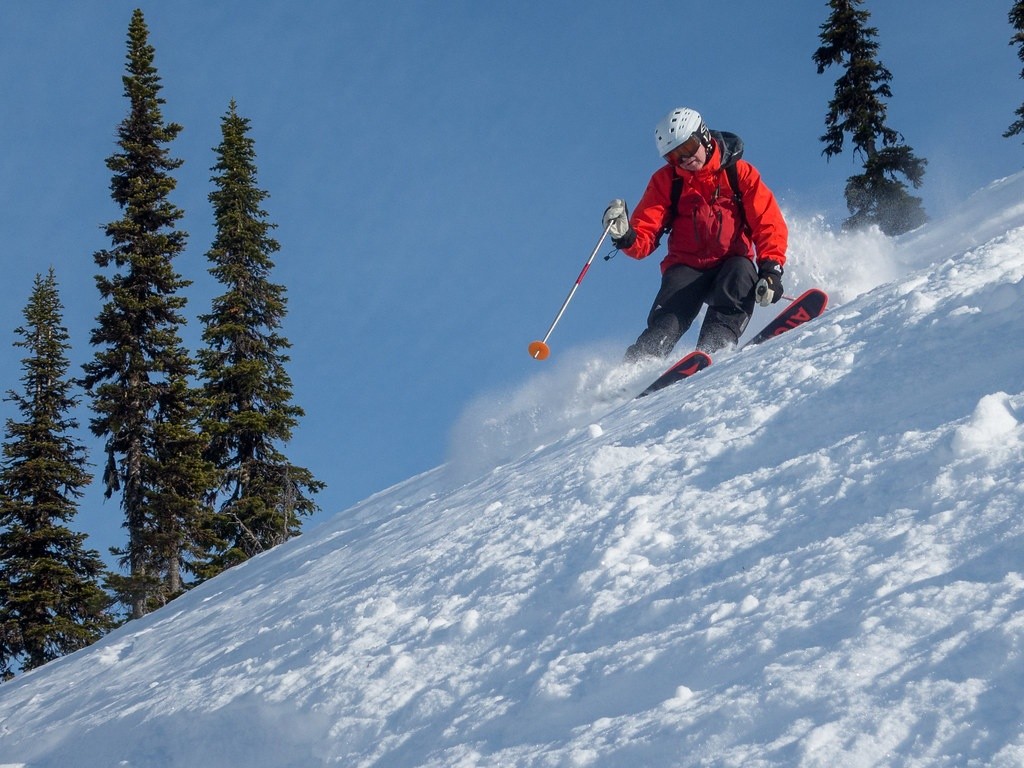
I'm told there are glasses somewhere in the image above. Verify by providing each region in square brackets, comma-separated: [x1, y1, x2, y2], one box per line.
[663, 133, 702, 167]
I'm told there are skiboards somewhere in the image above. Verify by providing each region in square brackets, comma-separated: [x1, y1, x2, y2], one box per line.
[634, 288, 829, 399]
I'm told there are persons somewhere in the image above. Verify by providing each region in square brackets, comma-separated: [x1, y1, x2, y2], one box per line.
[602, 108, 788, 402]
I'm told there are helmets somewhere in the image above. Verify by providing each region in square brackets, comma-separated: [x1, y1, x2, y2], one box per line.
[655, 107, 711, 157]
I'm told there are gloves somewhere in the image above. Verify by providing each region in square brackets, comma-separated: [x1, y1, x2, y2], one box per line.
[752, 259, 784, 306]
[602, 199, 637, 250]
